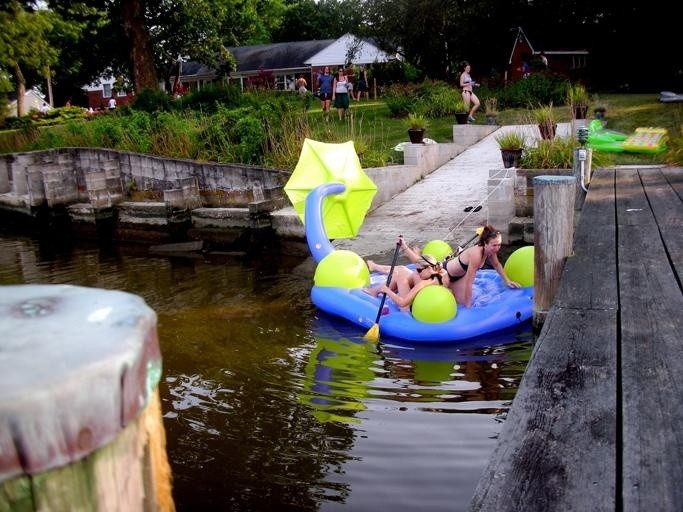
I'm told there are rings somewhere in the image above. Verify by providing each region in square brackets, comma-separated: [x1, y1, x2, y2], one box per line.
[511, 281, 513, 285]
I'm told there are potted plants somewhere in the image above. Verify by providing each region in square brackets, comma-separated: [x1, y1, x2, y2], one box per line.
[406, 112, 429, 143]
[492, 82, 590, 169]
[453, 100, 496, 125]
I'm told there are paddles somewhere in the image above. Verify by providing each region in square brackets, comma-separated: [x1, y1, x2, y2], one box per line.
[365, 234, 403, 340]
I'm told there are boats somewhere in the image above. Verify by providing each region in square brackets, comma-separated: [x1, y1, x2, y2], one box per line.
[282, 311, 537, 429]
[280, 132, 541, 344]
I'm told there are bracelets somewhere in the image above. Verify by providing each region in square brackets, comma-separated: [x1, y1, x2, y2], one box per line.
[402, 247, 409, 251]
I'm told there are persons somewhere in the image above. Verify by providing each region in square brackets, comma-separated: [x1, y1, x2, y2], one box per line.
[539, 52, 548, 67]
[355, 64, 369, 103]
[107, 96, 117, 111]
[38, 103, 47, 112]
[331, 66, 350, 121]
[315, 65, 335, 123]
[64, 96, 72, 107]
[360, 235, 451, 318]
[344, 62, 357, 101]
[517, 58, 531, 80]
[357, 66, 368, 99]
[459, 60, 480, 124]
[385, 224, 523, 311]
[296, 74, 307, 96]
[83, 107, 104, 116]
